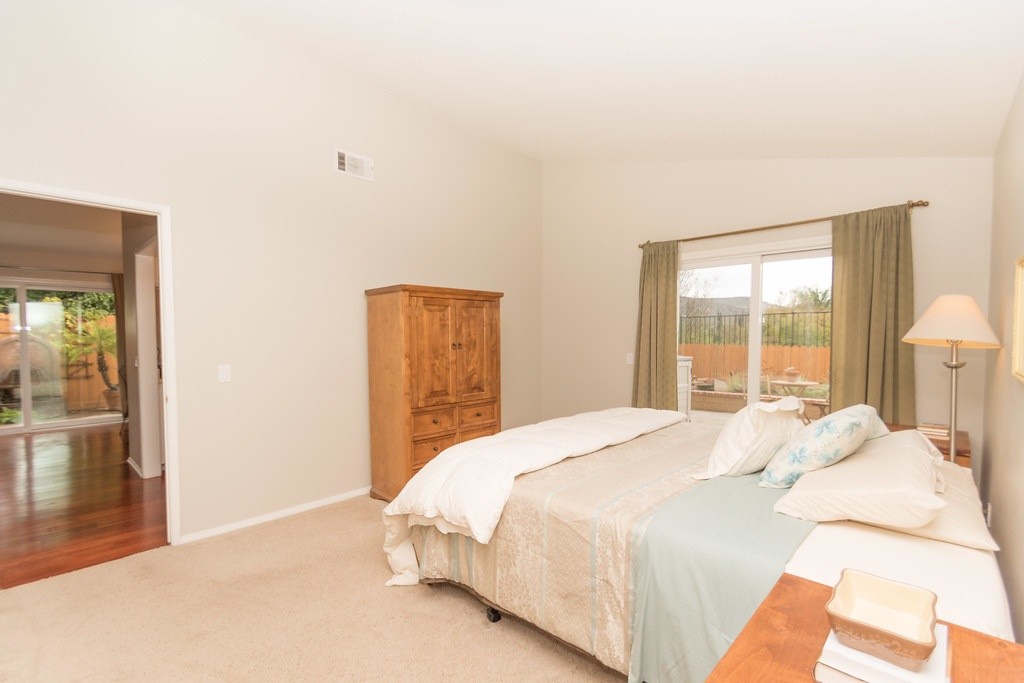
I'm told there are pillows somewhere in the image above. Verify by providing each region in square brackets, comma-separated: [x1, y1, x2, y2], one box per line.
[757, 404, 891, 489]
[691, 393, 805, 480]
[907, 460, 1001, 552]
[772, 430, 945, 530]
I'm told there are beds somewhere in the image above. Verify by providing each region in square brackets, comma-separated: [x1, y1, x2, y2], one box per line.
[380, 407, 1015, 683]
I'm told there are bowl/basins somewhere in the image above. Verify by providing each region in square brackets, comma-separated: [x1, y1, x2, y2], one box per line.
[824, 567, 938, 674]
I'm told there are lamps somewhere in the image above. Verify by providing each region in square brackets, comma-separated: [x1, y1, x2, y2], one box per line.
[900, 295, 1002, 464]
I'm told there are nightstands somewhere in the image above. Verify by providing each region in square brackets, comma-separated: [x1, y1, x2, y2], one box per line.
[886, 423, 970, 467]
[703, 573, 1024, 683]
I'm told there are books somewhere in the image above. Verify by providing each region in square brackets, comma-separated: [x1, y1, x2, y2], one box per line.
[916, 424, 949, 441]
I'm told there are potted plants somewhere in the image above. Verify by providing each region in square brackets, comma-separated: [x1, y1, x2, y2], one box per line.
[70, 321, 123, 411]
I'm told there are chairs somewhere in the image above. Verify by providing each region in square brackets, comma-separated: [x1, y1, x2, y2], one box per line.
[741, 372, 774, 403]
[813, 391, 830, 420]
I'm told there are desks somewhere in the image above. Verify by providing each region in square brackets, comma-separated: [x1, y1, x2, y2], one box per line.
[772, 381, 819, 425]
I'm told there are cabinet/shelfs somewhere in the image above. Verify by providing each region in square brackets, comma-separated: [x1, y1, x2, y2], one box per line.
[365, 284, 504, 502]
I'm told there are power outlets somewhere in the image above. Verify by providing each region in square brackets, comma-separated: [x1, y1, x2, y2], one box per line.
[986, 503, 991, 527]
